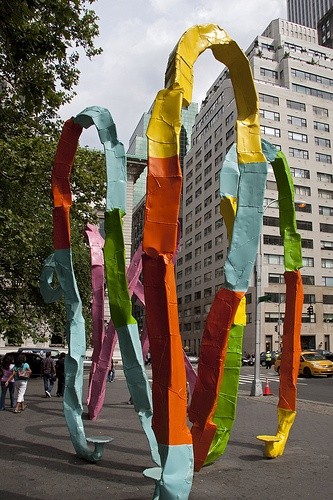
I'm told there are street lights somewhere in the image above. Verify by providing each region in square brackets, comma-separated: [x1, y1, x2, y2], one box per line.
[250, 197, 307, 396]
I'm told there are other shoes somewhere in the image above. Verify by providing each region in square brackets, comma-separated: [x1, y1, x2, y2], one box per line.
[14, 410, 21, 413]
[46, 396, 48, 398]
[45, 391, 51, 397]
[21, 405, 27, 411]
[11, 405, 16, 407]
[0, 407, 5, 411]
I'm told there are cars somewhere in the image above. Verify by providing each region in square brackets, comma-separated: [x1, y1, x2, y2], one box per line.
[0, 348, 62, 376]
[185, 352, 200, 363]
[275, 352, 333, 378]
[242, 353, 274, 366]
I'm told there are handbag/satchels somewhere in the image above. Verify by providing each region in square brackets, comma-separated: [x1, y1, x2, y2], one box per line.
[18, 363, 31, 379]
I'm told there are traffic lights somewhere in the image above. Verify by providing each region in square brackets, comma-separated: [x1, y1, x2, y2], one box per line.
[307, 306, 313, 315]
[275, 325, 277, 332]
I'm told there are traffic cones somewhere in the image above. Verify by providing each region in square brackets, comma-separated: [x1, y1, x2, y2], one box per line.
[263, 376, 273, 395]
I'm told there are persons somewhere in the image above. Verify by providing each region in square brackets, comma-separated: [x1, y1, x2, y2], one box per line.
[107, 359, 115, 383]
[265, 351, 272, 369]
[5, 355, 32, 413]
[41, 351, 56, 398]
[55, 352, 65, 397]
[244, 351, 255, 359]
[274, 352, 279, 359]
[0, 355, 16, 410]
